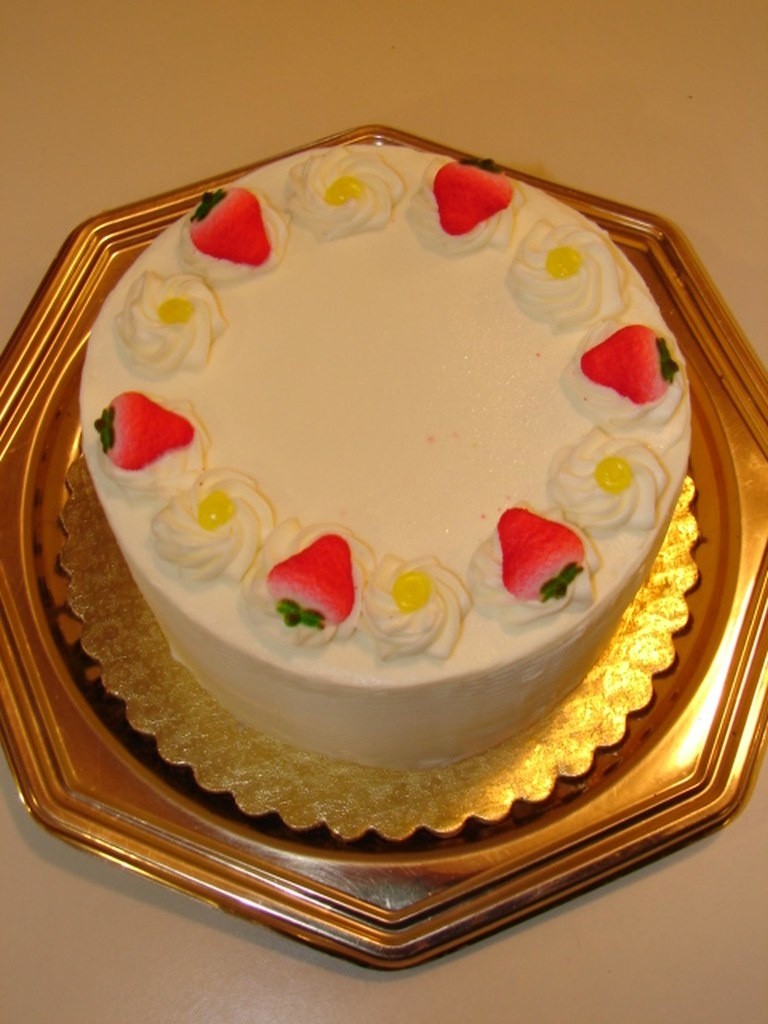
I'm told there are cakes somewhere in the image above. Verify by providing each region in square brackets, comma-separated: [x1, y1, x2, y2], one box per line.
[75, 143, 693, 773]
